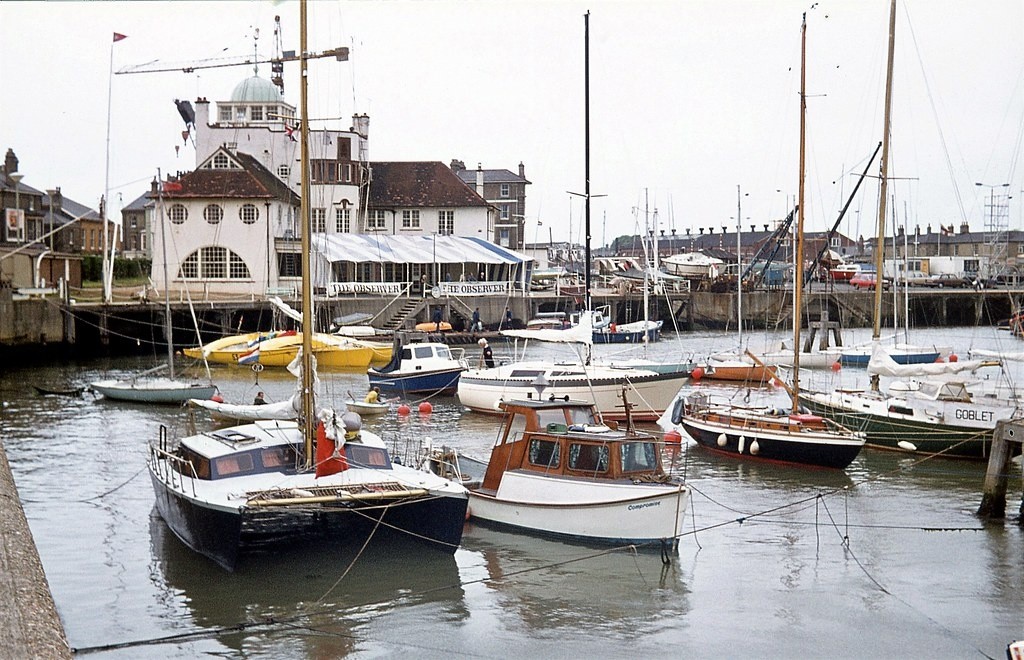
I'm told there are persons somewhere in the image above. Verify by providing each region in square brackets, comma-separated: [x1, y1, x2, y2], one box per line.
[467, 272, 480, 283]
[479, 272, 485, 281]
[421, 275, 427, 298]
[433, 306, 444, 333]
[364, 387, 385, 405]
[478, 338, 494, 368]
[254, 392, 268, 405]
[506, 307, 512, 330]
[470, 308, 482, 334]
[445, 273, 452, 282]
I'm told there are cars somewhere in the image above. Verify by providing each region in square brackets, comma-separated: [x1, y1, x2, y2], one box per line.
[925, 272, 969, 290]
[899, 271, 935, 287]
[849, 274, 891, 292]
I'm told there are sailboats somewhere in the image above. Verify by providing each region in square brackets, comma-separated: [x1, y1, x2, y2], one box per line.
[88, 167, 225, 406]
[146, 2, 472, 553]
[184, 0, 1024, 550]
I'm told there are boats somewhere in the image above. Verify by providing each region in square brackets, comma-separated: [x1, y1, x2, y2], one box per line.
[338, 324, 377, 337]
[413, 321, 453, 332]
[331, 312, 374, 328]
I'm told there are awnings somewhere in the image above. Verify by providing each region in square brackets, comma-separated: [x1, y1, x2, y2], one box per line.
[613, 267, 684, 295]
[532, 268, 568, 284]
[310, 233, 536, 296]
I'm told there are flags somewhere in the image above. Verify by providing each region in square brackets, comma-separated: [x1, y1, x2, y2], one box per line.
[238, 347, 259, 365]
[161, 180, 183, 192]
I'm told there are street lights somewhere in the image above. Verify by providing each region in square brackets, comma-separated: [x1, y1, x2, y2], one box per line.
[431, 230, 436, 288]
[9, 171, 26, 246]
[46, 190, 56, 254]
[974, 182, 1010, 280]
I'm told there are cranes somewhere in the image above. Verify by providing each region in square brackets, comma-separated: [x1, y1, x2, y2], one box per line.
[115, 17, 351, 99]
